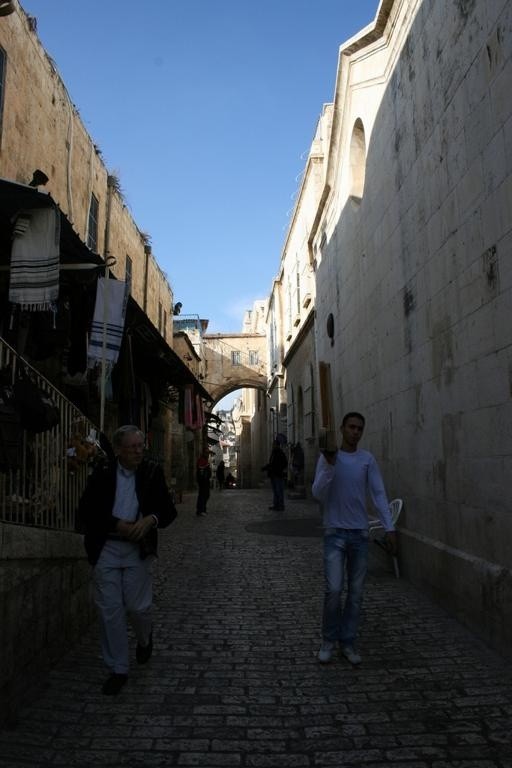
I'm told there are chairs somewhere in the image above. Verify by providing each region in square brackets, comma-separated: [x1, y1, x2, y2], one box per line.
[368, 498, 405, 580]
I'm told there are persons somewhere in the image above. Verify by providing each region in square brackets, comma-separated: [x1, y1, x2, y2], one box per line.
[312, 411, 398, 665]
[225, 472, 234, 487]
[260, 440, 288, 512]
[195, 447, 211, 515]
[75, 425, 177, 696]
[216, 459, 225, 490]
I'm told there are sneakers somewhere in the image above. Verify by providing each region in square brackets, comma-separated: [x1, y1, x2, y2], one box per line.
[344, 647, 363, 666]
[101, 673, 126, 695]
[319, 640, 332, 661]
[135, 624, 153, 666]
